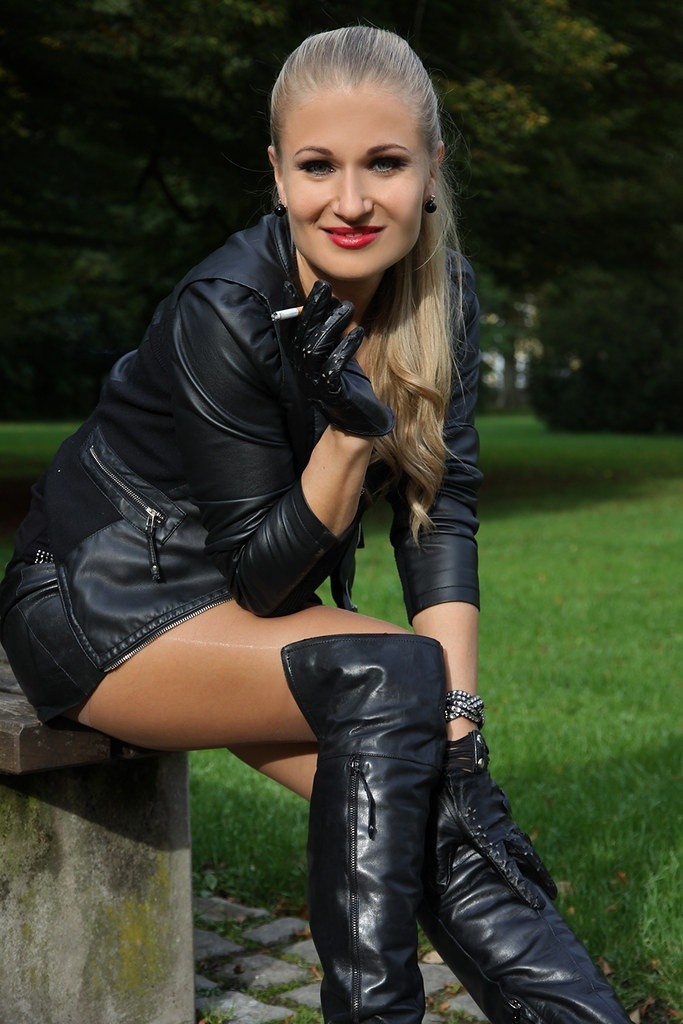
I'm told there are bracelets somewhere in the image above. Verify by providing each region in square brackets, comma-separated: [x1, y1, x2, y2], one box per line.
[443, 689, 485, 730]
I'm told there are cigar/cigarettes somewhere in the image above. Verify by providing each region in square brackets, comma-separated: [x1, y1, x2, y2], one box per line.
[271, 305, 306, 323]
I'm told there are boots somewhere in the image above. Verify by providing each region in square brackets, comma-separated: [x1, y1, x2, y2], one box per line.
[281, 632, 446, 1024]
[416, 839, 636, 1024]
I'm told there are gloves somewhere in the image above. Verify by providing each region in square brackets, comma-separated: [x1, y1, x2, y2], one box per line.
[435, 731, 557, 911]
[281, 280, 395, 436]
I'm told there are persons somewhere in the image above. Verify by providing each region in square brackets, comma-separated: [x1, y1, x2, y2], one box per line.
[0, 23, 635, 1024]
